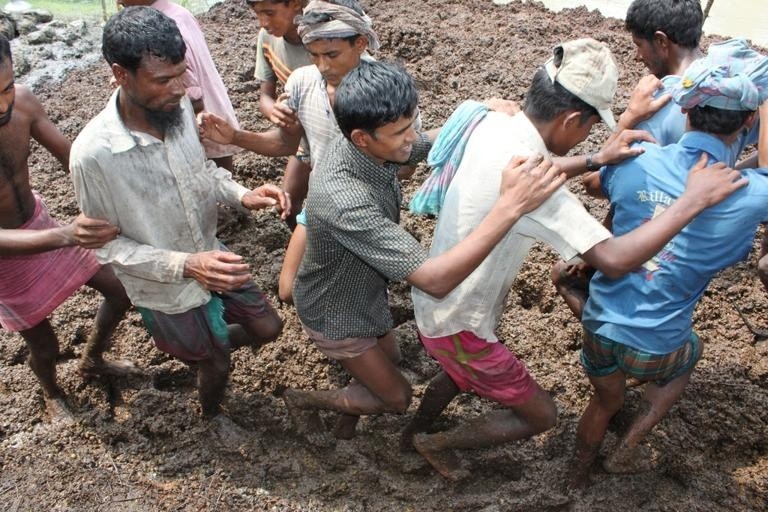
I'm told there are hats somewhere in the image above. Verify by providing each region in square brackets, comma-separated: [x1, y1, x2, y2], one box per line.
[545, 38, 619, 135]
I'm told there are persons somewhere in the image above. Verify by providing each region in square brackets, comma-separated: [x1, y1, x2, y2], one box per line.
[113, 0, 245, 177]
[281, 64, 570, 453]
[549, 0, 765, 325]
[0, 32, 150, 425]
[66, 9, 294, 454]
[565, 68, 768, 483]
[196, 1, 425, 304]
[396, 40, 753, 482]
[247, 0, 378, 231]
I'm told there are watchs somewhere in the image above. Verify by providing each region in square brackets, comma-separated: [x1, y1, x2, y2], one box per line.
[585, 153, 596, 173]
